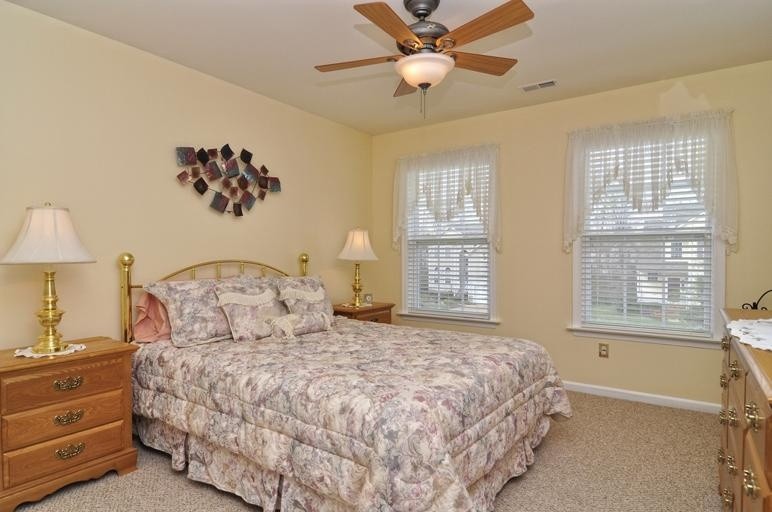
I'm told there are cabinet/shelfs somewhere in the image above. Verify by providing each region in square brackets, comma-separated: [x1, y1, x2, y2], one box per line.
[714, 306, 772, 510]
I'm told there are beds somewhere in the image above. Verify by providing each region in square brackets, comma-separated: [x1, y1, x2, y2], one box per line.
[117, 248, 567, 512]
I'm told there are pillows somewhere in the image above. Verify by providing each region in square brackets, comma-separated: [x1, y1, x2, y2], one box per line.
[135, 271, 340, 349]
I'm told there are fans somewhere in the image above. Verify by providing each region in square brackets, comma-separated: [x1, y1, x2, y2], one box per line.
[311, 0, 536, 99]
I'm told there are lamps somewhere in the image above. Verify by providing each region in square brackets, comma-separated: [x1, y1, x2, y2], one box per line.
[2, 201, 98, 356]
[337, 227, 379, 309]
[392, 54, 457, 120]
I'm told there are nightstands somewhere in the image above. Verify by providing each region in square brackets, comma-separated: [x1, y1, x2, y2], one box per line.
[2, 336, 147, 512]
[330, 301, 397, 324]
